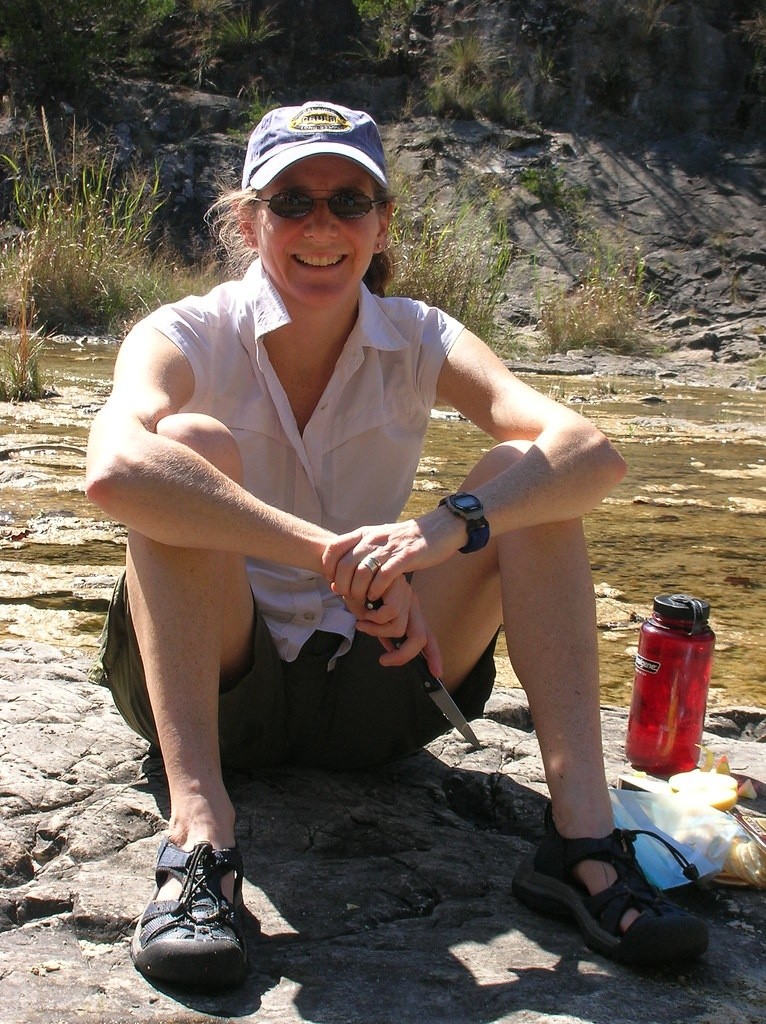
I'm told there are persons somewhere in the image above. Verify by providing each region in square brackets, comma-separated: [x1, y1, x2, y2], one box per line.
[85, 100, 711, 991]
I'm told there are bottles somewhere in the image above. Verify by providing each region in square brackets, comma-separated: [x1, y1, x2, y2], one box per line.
[625, 593, 716, 772]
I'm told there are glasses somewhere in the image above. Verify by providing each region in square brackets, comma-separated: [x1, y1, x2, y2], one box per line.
[252, 191, 385, 219]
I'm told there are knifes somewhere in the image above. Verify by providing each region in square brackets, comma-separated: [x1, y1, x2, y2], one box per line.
[366, 599, 484, 752]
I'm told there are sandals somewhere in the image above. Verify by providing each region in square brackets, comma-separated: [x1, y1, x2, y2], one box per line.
[130, 838, 247, 984]
[511, 801, 709, 964]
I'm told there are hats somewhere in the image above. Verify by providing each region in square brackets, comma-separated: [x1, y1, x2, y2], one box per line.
[242, 103, 387, 204]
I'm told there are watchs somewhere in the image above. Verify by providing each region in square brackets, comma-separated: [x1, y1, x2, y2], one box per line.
[437, 491, 490, 554]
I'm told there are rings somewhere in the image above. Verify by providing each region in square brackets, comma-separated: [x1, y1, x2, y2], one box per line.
[361, 554, 382, 576]
